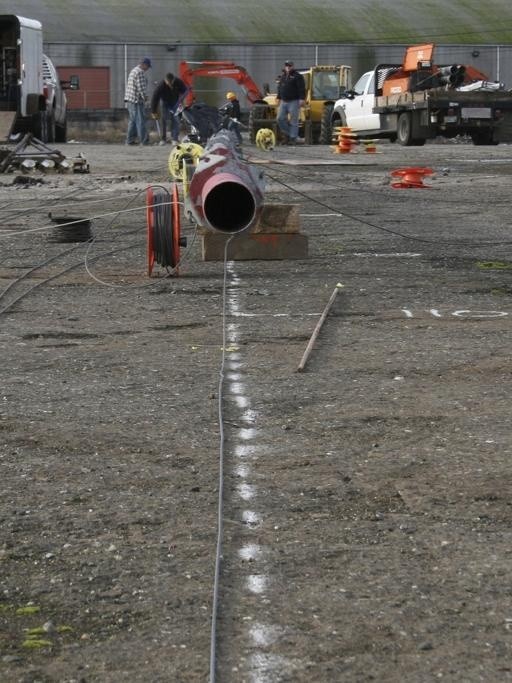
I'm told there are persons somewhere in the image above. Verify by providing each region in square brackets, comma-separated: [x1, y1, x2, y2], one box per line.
[124, 58, 154, 146]
[275, 59, 307, 145]
[221, 92, 242, 142]
[151, 72, 187, 146]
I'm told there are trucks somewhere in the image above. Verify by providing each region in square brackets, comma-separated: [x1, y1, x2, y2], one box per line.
[0, 10, 82, 145]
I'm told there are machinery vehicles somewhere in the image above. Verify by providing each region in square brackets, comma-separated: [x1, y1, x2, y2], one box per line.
[173, 54, 357, 150]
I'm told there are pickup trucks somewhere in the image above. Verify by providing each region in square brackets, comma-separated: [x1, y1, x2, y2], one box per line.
[324, 63, 512, 148]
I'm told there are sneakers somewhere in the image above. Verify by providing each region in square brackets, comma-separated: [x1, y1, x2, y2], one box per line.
[158, 136, 179, 147]
[277, 134, 299, 146]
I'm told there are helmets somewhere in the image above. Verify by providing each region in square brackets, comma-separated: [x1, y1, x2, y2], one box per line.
[225, 92, 237, 100]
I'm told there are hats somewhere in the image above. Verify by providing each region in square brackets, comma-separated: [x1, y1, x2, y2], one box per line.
[139, 56, 152, 69]
[284, 59, 294, 67]
